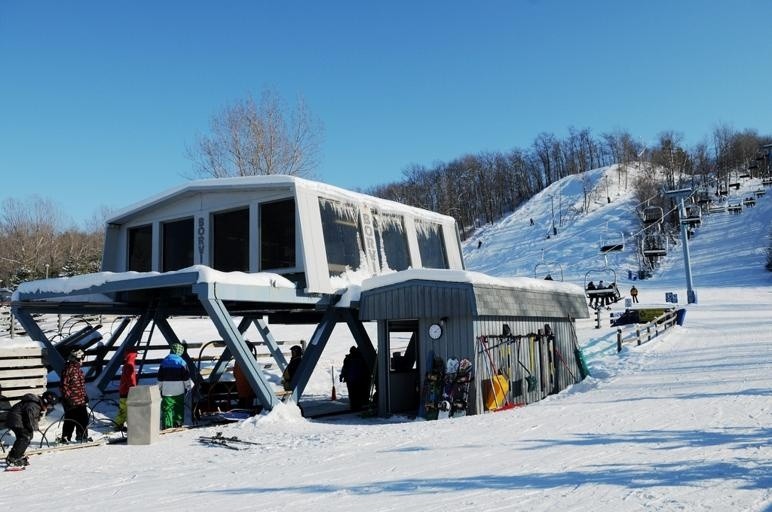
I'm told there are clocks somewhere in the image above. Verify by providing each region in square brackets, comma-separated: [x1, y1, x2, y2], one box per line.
[428, 324, 442, 340]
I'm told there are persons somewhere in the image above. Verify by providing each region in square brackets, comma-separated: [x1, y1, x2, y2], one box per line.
[544, 274, 553, 280]
[339, 347, 372, 409]
[6, 391, 56, 467]
[728, 197, 755, 215]
[59, 348, 93, 443]
[235, 341, 257, 409]
[157, 343, 195, 430]
[115, 348, 137, 431]
[630, 285, 639, 303]
[588, 280, 620, 307]
[689, 213, 695, 229]
[283, 347, 304, 417]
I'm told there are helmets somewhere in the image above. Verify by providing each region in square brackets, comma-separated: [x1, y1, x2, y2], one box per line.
[169, 343, 185, 356]
[42, 391, 59, 407]
[70, 344, 90, 362]
[290, 346, 302, 355]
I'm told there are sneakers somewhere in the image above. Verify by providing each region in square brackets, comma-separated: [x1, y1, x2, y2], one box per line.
[62, 435, 89, 444]
[6, 456, 30, 466]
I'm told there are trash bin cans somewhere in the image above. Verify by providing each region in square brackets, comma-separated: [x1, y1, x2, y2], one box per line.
[127, 386, 161, 446]
[665, 292, 677, 303]
